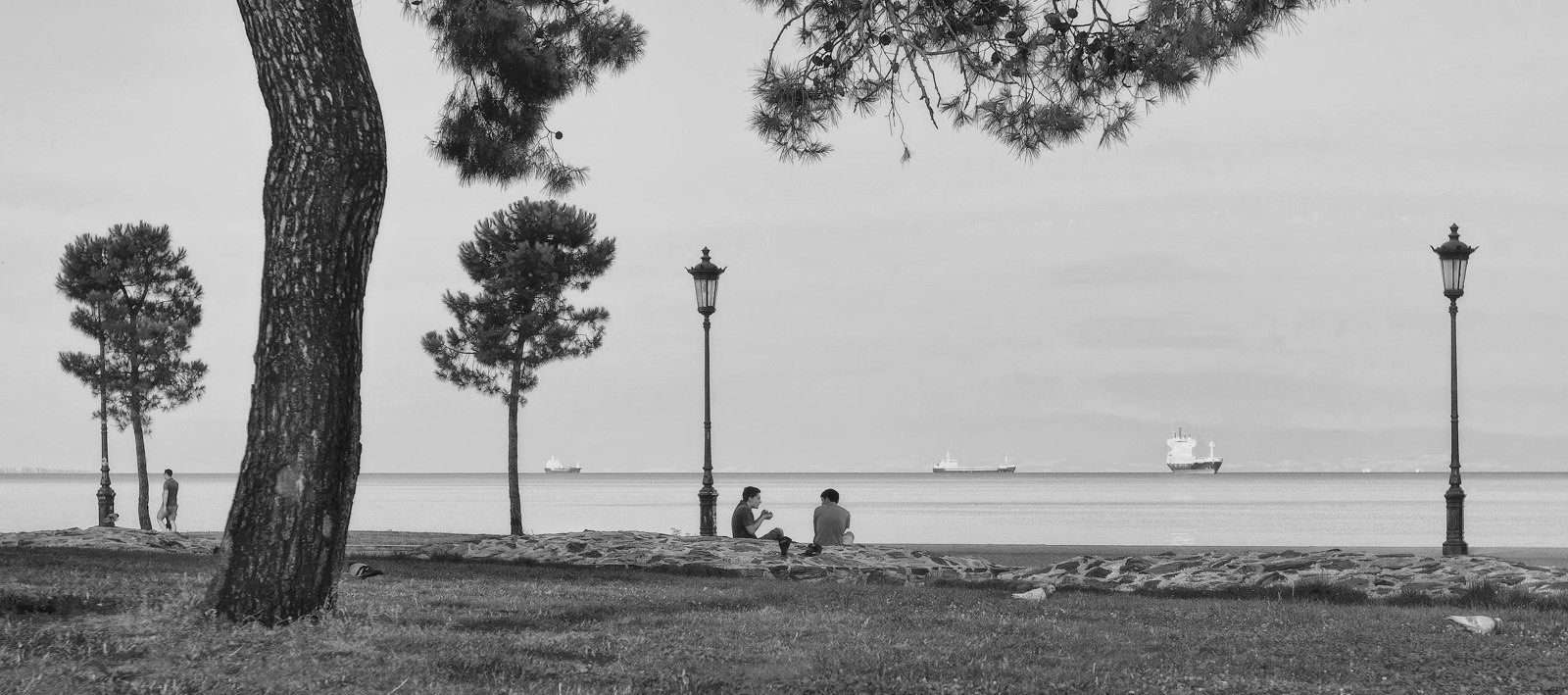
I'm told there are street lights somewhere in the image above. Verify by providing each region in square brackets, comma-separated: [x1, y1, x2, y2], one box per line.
[1430, 223, 1479, 556]
[685, 246, 727, 538]
[92, 300, 116, 527]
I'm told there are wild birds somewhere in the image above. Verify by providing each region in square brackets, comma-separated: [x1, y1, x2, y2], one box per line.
[349, 561, 383, 578]
[1013, 584, 1056, 601]
[1447, 616, 1503, 636]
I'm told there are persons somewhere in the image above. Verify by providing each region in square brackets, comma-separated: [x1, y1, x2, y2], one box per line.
[156, 469, 179, 532]
[812, 489, 854, 546]
[732, 486, 784, 540]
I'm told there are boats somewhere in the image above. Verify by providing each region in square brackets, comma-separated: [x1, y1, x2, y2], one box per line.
[1167, 425, 1224, 474]
[933, 451, 1016, 473]
[545, 456, 581, 473]
